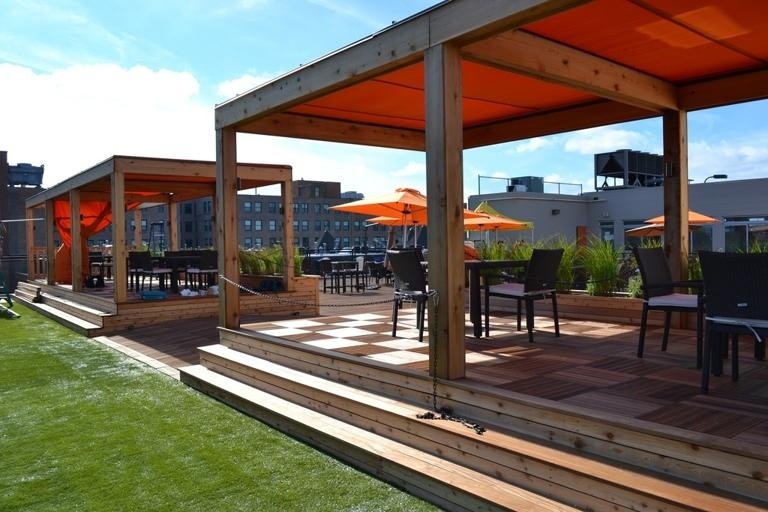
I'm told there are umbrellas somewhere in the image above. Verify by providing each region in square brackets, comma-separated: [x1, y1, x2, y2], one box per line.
[325, 186, 491, 248]
[366, 217, 426, 249]
[623, 222, 702, 237]
[643, 209, 722, 225]
[464, 211, 526, 259]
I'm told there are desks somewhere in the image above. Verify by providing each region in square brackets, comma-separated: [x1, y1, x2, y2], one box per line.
[387, 260, 534, 339]
[710, 330, 765, 379]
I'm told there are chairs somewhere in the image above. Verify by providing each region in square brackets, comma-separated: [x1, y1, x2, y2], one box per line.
[698, 250, 768, 394]
[318, 255, 394, 294]
[486, 248, 564, 342]
[89, 250, 218, 293]
[414, 248, 425, 261]
[633, 247, 702, 369]
[386, 249, 429, 342]
